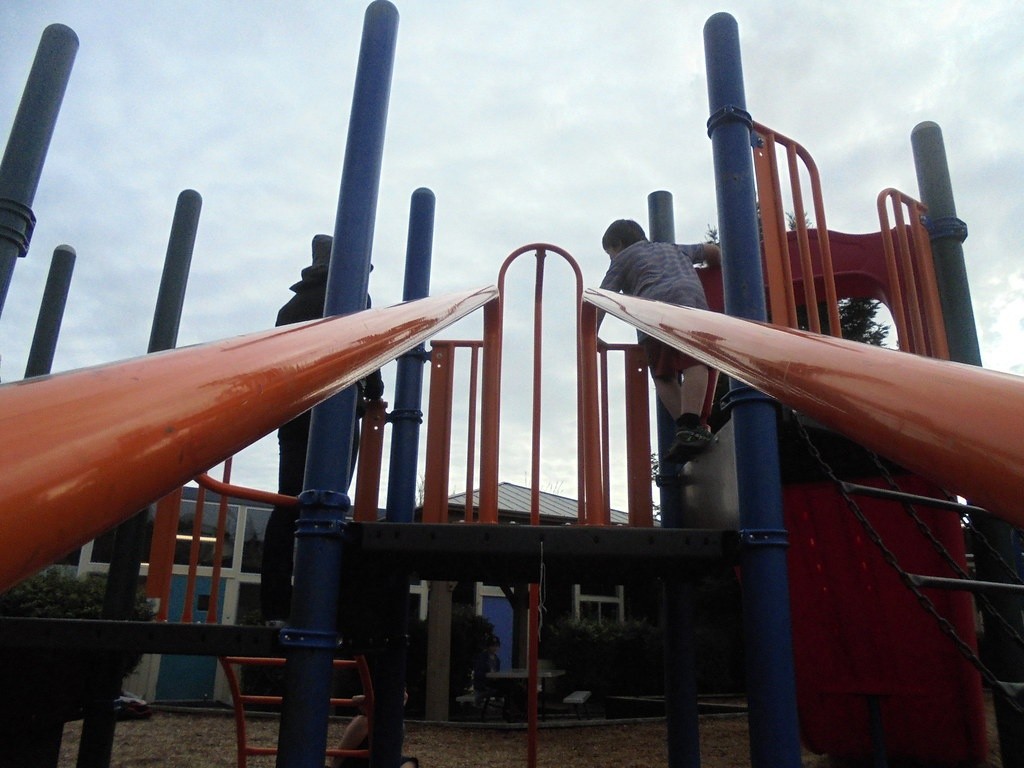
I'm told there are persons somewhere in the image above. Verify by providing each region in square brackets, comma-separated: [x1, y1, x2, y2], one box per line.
[593, 219, 721, 458]
[263, 235, 386, 627]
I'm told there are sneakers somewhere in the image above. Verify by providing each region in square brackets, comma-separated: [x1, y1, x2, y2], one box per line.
[661, 438, 684, 464]
[675, 426, 715, 449]
